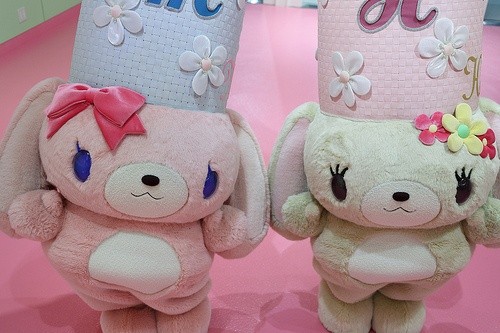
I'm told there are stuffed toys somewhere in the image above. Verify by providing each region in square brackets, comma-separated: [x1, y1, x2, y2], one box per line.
[269, 1, 499, 332]
[0, 1, 271, 332]
